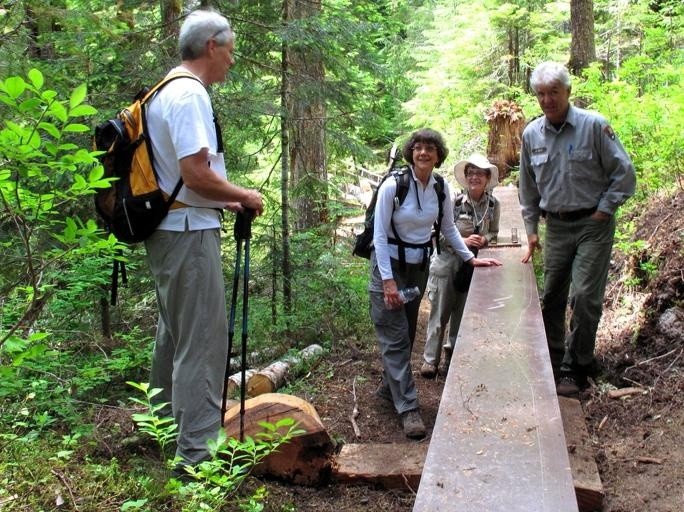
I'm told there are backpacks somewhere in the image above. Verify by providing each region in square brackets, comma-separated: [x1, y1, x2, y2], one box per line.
[352, 166, 445, 260]
[94, 73, 224, 243]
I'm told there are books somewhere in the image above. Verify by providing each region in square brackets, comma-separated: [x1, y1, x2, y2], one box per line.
[487, 236, 522, 247]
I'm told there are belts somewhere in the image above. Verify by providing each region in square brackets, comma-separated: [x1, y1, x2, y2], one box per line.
[539, 205, 598, 222]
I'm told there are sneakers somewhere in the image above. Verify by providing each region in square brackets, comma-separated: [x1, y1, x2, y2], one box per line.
[421, 361, 437, 375]
[376, 384, 392, 401]
[570, 359, 596, 391]
[400, 408, 427, 437]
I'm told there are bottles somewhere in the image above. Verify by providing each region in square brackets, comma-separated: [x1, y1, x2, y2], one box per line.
[384, 287, 420, 311]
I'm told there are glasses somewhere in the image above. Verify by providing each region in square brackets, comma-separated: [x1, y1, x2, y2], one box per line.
[411, 145, 437, 152]
[465, 171, 487, 177]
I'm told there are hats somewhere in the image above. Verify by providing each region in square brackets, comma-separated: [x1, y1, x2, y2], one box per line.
[454, 153, 499, 190]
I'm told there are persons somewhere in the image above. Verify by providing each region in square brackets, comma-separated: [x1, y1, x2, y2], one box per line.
[417, 150, 503, 380]
[517, 58, 638, 400]
[132, 8, 268, 481]
[363, 126, 504, 442]
